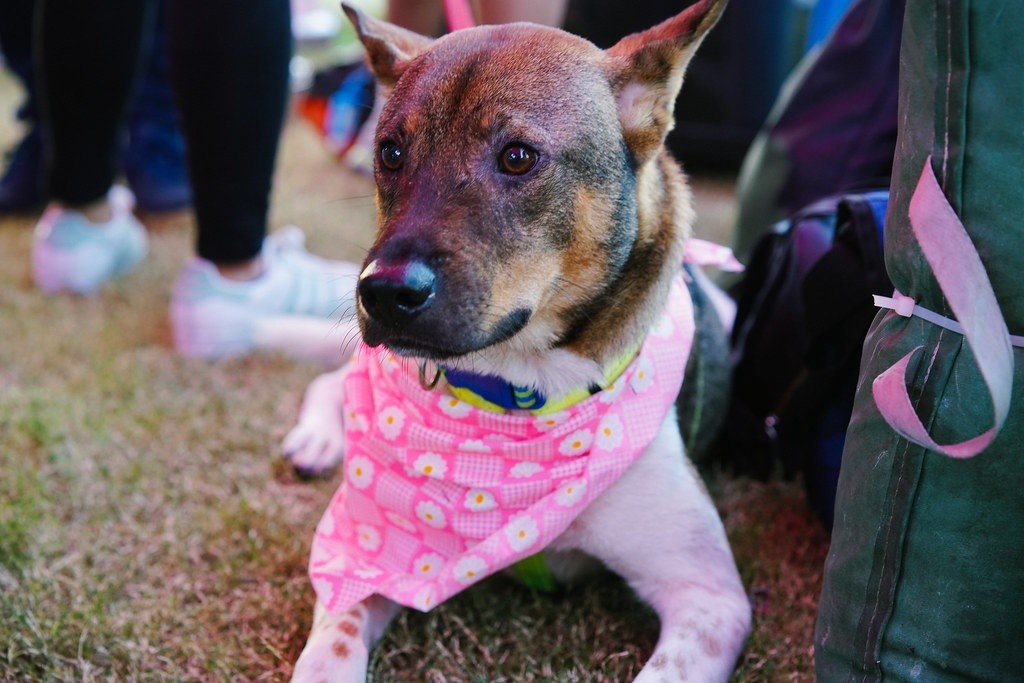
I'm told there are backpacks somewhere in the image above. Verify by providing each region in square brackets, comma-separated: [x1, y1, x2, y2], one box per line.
[717, 187, 889, 530]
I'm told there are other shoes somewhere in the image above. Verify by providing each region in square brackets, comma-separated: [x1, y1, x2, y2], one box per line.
[34, 184, 148, 294]
[169, 219, 365, 362]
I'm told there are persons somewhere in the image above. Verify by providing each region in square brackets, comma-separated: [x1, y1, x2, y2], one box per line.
[342, 2, 565, 174]
[1, 1, 372, 353]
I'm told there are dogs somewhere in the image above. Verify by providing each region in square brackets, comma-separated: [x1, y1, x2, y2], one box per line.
[279, 0, 758, 683]
